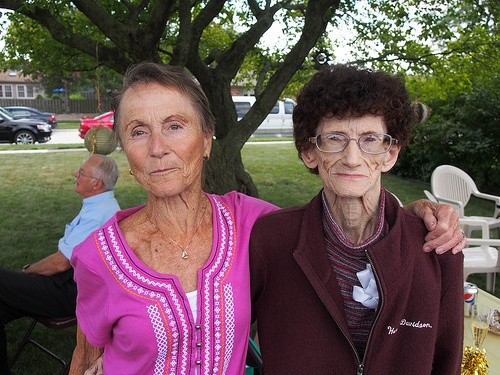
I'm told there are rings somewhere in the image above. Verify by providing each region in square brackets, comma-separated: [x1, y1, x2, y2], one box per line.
[457, 228, 466, 238]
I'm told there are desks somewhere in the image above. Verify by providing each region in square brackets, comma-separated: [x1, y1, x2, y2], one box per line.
[462, 282, 500, 375]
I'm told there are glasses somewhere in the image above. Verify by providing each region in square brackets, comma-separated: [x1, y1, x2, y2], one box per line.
[77, 170, 107, 186]
[308, 131, 398, 155]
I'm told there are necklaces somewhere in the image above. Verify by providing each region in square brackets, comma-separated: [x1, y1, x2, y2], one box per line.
[146, 200, 208, 259]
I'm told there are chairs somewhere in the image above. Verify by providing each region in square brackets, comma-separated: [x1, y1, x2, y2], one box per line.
[9, 314, 77, 375]
[424, 164, 500, 239]
[423, 189, 500, 295]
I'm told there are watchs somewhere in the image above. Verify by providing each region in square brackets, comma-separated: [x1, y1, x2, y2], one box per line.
[22, 264, 30, 271]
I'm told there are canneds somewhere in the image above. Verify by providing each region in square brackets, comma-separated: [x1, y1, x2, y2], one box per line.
[464, 283, 478, 316]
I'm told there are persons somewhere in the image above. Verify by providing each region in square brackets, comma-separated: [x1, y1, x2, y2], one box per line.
[84, 69, 464, 375]
[0, 155, 122, 375]
[69, 61, 465, 375]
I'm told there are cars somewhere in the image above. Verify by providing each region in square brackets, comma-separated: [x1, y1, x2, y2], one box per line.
[79, 110, 115, 139]
[0, 107, 52, 145]
[3, 106, 57, 129]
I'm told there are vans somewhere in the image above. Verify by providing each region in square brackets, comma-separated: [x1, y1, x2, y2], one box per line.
[232, 96, 298, 138]
